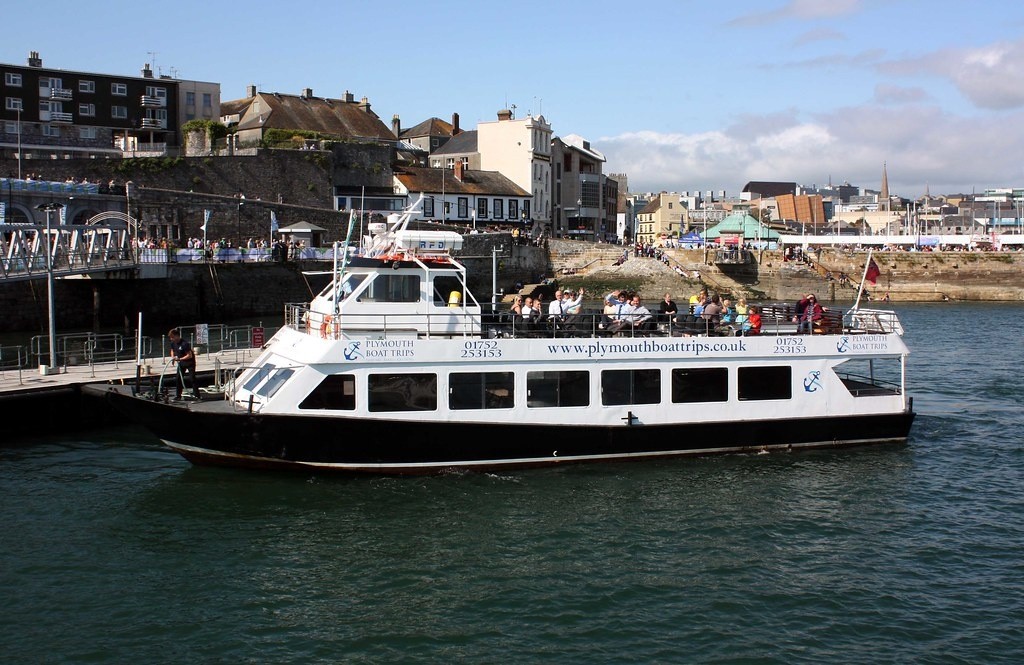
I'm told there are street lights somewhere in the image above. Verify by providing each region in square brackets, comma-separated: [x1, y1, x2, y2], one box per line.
[577, 199, 582, 240]
[34, 202, 65, 370]
[235, 187, 245, 246]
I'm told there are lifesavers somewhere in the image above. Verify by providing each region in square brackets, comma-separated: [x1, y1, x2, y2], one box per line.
[320, 315, 339, 340]
[378, 256, 403, 260]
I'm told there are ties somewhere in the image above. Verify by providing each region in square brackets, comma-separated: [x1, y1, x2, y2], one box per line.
[618, 305, 622, 319]
[559, 303, 563, 318]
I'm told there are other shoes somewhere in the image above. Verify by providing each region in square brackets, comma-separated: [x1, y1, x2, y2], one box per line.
[171, 397, 180, 402]
[191, 397, 202, 404]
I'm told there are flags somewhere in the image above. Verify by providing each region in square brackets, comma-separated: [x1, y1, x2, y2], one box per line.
[200, 210, 211, 233]
[866, 258, 880, 284]
[271, 211, 279, 232]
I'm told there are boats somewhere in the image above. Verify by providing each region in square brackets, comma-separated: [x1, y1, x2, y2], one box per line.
[72, 188, 919, 480]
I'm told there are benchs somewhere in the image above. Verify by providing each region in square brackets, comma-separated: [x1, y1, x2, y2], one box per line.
[811, 310, 842, 332]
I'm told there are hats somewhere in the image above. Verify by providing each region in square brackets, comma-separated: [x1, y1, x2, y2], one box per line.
[563, 290, 569, 294]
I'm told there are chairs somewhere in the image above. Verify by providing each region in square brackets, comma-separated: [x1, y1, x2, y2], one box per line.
[502, 309, 655, 337]
[665, 313, 706, 336]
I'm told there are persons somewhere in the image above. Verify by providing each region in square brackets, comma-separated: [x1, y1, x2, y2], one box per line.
[511, 286, 821, 337]
[827, 270, 890, 302]
[168, 329, 201, 401]
[139, 209, 1024, 264]
[25, 172, 245, 208]
